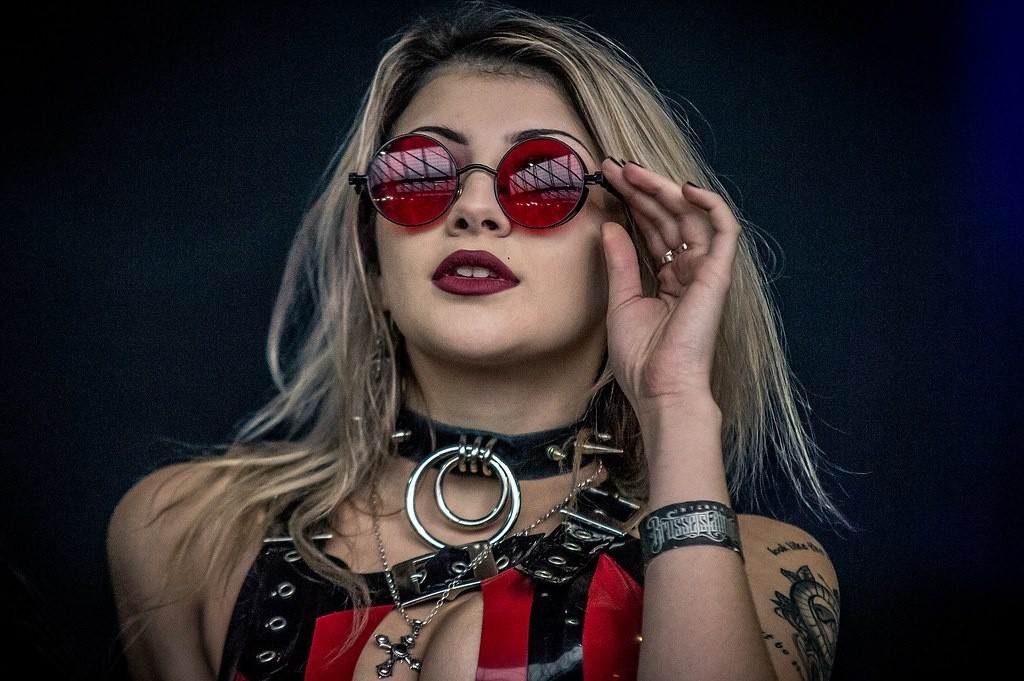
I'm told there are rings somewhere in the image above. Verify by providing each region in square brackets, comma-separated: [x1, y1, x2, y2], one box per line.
[657, 251, 673, 270]
[671, 243, 687, 257]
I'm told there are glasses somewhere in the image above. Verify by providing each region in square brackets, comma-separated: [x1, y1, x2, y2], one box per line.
[348, 133, 626, 230]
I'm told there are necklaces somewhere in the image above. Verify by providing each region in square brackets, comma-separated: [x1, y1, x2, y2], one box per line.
[386, 405, 624, 549]
[369, 459, 603, 679]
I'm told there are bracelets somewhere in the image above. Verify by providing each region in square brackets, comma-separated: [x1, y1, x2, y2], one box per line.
[638, 500, 745, 574]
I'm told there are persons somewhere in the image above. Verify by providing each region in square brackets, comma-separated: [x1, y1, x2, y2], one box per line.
[106, 0, 853, 681]
